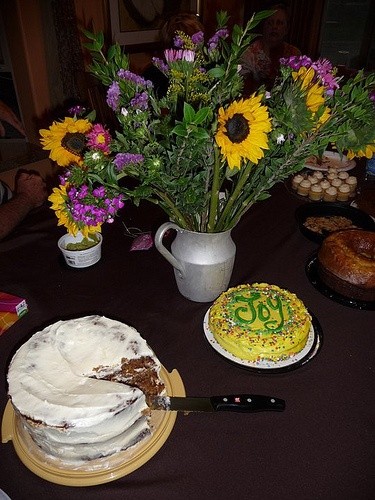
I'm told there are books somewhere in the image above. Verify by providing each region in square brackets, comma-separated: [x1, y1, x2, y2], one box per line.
[0, 291, 28, 337]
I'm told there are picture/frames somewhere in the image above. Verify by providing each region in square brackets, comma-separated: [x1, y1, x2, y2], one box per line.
[102, 0, 204, 54]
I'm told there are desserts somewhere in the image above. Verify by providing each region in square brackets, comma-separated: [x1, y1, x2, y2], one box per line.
[291, 167, 357, 202]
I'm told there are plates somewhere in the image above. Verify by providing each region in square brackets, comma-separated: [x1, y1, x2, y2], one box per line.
[304, 150, 356, 172]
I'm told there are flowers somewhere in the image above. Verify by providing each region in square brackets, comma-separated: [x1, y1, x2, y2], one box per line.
[39, 7, 375, 251]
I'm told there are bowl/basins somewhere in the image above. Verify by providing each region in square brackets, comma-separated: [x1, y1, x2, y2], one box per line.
[57, 229, 103, 268]
[292, 202, 375, 254]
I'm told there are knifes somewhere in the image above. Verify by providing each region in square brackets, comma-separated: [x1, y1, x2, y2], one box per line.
[146, 394, 287, 414]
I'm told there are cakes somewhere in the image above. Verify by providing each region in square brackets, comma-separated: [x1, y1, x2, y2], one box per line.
[207, 282, 311, 362]
[7, 315, 167, 462]
[316, 230, 375, 291]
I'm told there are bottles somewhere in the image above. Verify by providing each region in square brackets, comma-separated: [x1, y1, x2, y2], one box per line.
[364, 155, 375, 198]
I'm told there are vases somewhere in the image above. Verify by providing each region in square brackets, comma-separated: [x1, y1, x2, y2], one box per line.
[155, 192, 240, 303]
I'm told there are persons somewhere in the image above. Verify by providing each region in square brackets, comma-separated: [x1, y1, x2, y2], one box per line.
[0, 170, 49, 237]
[144, 16, 221, 127]
[241, 4, 307, 81]
